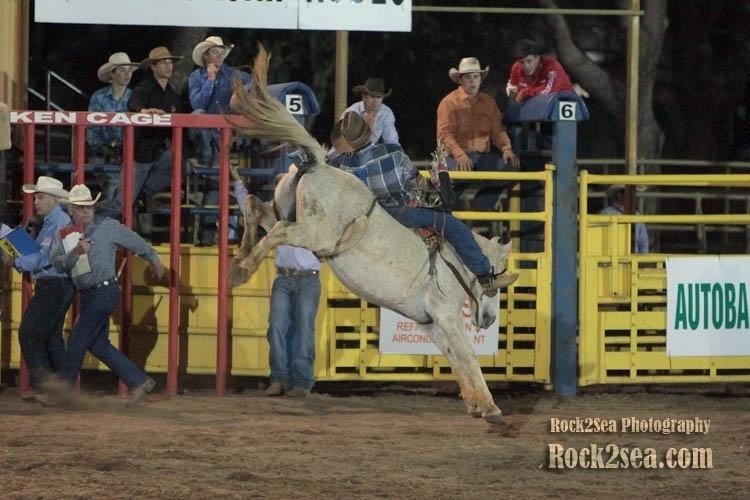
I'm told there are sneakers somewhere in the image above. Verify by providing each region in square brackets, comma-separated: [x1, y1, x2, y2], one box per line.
[125, 377, 155, 409]
[265, 381, 285, 396]
[21, 390, 74, 407]
[484, 271, 520, 299]
[286, 386, 311, 398]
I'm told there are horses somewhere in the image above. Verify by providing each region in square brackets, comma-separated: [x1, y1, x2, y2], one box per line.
[219, 42, 513, 426]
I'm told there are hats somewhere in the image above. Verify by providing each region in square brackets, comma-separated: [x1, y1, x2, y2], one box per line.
[330, 111, 376, 153]
[22, 175, 68, 200]
[139, 46, 185, 74]
[449, 56, 492, 84]
[58, 183, 102, 207]
[97, 51, 141, 84]
[352, 76, 394, 100]
[192, 35, 235, 67]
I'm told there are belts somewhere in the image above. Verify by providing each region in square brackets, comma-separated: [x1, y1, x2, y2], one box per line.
[277, 267, 320, 278]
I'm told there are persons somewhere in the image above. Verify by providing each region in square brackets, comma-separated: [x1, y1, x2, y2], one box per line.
[32, 184, 166, 407]
[0, 177, 73, 400]
[84, 35, 650, 297]
[227, 162, 321, 397]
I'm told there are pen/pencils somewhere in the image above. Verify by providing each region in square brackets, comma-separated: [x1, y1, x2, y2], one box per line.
[78, 237, 95, 245]
